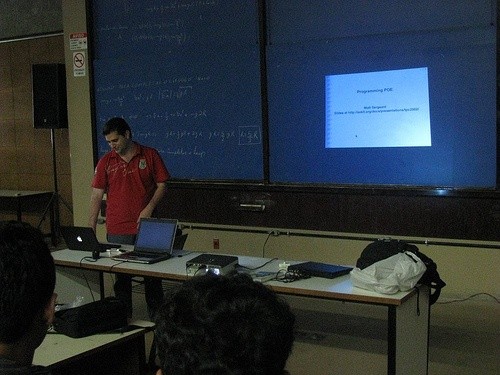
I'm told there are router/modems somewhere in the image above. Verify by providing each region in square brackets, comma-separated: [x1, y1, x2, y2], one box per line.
[186, 254, 239, 276]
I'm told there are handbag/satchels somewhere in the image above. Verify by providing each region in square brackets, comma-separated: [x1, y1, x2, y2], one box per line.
[349, 250, 426, 294]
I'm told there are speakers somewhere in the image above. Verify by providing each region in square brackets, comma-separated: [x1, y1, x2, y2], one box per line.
[31, 63, 68, 129]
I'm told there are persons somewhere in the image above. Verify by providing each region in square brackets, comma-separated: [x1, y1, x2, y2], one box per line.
[0, 219, 64, 375]
[88, 116, 172, 323]
[156, 266, 299, 375]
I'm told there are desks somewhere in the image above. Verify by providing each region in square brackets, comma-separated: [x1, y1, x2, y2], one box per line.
[32, 317, 156, 374]
[49, 240, 432, 375]
[0, 189, 56, 246]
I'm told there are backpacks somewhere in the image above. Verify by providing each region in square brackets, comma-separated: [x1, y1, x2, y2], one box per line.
[356, 239, 446, 304]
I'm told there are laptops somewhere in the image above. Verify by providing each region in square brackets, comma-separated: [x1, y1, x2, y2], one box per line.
[288, 261, 353, 279]
[60, 218, 178, 263]
